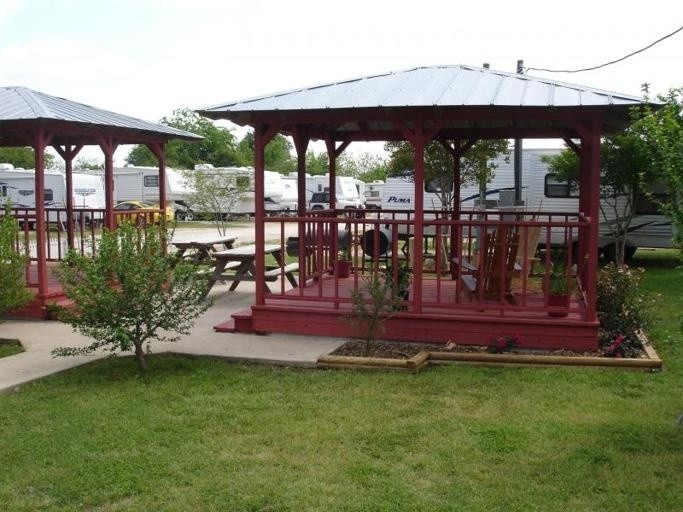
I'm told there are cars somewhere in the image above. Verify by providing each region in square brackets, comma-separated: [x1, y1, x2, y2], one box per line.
[113, 202, 173, 226]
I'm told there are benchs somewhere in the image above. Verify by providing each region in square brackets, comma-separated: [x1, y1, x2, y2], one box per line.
[182, 255, 300, 281]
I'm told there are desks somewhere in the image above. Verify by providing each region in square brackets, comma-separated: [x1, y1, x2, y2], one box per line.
[197, 244, 298, 302]
[171, 236, 238, 284]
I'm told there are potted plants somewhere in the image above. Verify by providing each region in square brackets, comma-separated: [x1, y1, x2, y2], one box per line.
[333, 245, 351, 278]
[542, 260, 576, 316]
[385, 260, 410, 311]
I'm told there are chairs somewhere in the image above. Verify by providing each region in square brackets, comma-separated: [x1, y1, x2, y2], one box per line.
[452, 230, 523, 312]
